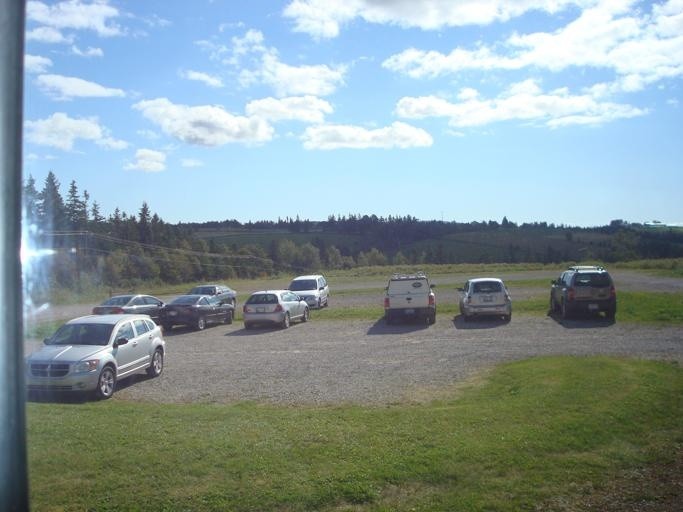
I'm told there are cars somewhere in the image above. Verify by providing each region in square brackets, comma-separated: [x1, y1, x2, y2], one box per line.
[90, 292, 167, 325]
[157, 293, 235, 331]
[188, 285, 237, 308]
[241, 289, 311, 330]
[453, 277, 513, 322]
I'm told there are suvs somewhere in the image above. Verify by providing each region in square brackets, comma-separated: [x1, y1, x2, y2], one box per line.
[383, 271, 438, 323]
[25, 312, 169, 402]
[549, 263, 619, 323]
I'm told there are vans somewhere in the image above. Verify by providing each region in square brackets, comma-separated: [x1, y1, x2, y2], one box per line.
[286, 273, 330, 310]
